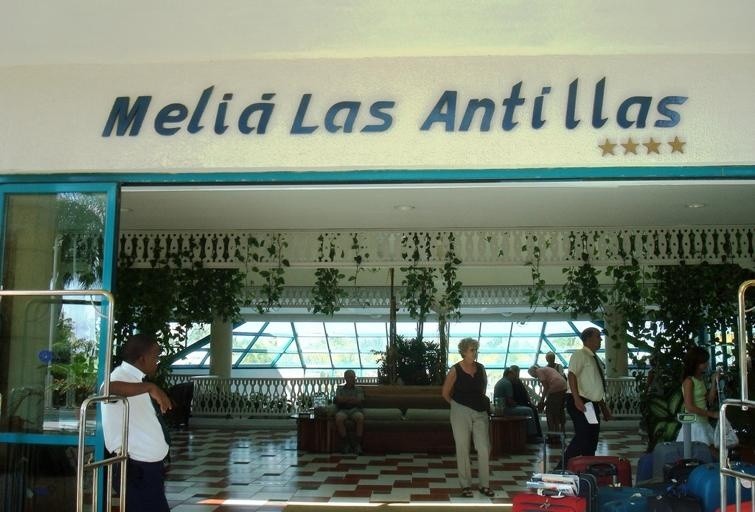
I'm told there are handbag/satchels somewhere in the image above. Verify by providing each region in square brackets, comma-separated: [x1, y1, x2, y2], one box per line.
[713, 416, 740, 450]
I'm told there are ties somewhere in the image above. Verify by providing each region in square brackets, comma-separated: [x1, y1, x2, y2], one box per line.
[594, 354, 609, 397]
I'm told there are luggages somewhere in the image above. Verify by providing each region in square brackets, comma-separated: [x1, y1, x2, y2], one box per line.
[566, 451, 634, 489]
[648, 489, 705, 512]
[594, 481, 656, 512]
[688, 458, 755, 512]
[526, 470, 600, 512]
[506, 491, 588, 512]
[636, 453, 653, 488]
[651, 438, 713, 487]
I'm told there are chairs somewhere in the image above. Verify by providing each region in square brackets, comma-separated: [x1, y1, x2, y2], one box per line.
[153, 382, 195, 431]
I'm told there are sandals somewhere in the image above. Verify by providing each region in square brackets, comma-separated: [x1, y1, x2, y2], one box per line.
[477, 485, 495, 497]
[462, 486, 473, 499]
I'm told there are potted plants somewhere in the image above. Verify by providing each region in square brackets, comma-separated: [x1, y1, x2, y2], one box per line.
[370, 328, 451, 385]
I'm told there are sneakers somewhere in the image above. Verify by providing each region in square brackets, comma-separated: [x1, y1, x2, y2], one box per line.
[526, 432, 567, 444]
[340, 444, 363, 455]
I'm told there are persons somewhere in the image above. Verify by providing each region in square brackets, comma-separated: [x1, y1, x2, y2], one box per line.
[648, 362, 657, 384]
[553, 329, 615, 470]
[334, 370, 365, 455]
[99, 335, 173, 511]
[676, 346, 721, 458]
[441, 339, 494, 498]
[494, 352, 568, 443]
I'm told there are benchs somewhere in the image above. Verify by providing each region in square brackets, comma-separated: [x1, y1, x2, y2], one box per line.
[290, 384, 533, 455]
